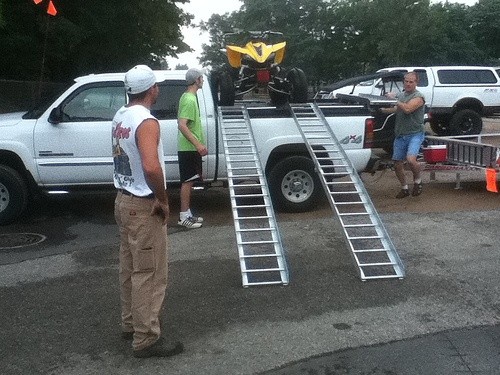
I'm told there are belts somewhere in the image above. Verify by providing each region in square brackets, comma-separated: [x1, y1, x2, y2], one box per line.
[119, 188, 154, 198]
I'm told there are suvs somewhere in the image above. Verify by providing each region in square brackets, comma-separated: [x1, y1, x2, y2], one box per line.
[314, 66, 500, 139]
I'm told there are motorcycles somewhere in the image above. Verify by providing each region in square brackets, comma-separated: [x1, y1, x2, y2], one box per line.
[211, 28, 308, 104]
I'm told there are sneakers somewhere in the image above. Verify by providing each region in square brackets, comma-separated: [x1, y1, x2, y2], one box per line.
[122, 331, 184, 357]
[412, 181, 423, 197]
[396, 188, 410, 199]
[177, 218, 202, 229]
[187, 216, 204, 223]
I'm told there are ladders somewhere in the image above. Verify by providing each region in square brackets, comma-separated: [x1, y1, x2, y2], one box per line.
[217, 103, 291, 288]
[425, 132, 500, 171]
[289, 101, 408, 282]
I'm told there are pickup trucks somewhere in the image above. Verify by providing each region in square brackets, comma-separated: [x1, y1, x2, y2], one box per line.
[0, 70, 375, 228]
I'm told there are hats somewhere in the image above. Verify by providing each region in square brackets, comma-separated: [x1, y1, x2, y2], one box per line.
[124, 65, 166, 94]
[186, 68, 205, 84]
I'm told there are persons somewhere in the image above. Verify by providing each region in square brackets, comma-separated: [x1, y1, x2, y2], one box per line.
[111, 65, 183, 357]
[177, 69, 209, 229]
[380, 72, 425, 199]
